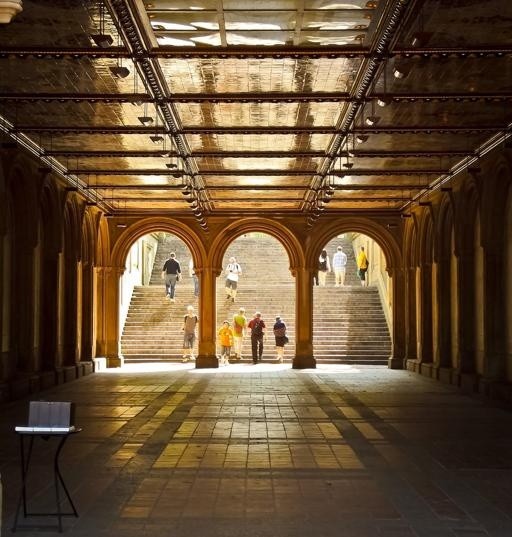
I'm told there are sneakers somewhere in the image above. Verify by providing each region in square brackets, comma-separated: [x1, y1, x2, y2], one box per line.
[182, 357, 188, 362]
[227, 293, 231, 298]
[189, 356, 197, 359]
[232, 296, 235, 301]
[222, 354, 284, 365]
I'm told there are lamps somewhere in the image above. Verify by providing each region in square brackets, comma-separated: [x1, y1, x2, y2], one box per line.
[116, 200, 128, 228]
[2, 107, 79, 192]
[365, 78, 380, 127]
[306, 102, 367, 231]
[129, 58, 147, 107]
[438, 157, 453, 193]
[103, 189, 113, 217]
[137, 86, 153, 127]
[392, 15, 409, 79]
[377, 59, 391, 109]
[466, 134, 482, 173]
[149, 94, 210, 235]
[91, 2, 114, 48]
[86, 174, 97, 205]
[410, 0, 432, 47]
[108, 34, 130, 79]
[386, 173, 431, 231]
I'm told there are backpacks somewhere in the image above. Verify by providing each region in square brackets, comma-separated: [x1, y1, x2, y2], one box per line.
[251, 319, 262, 335]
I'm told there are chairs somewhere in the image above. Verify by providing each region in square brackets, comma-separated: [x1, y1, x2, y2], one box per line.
[12, 401, 83, 533]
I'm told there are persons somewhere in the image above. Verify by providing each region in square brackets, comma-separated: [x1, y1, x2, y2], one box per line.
[189, 258, 199, 297]
[333, 246, 347, 287]
[273, 316, 289, 364]
[233, 308, 247, 360]
[318, 249, 331, 287]
[357, 246, 369, 287]
[247, 312, 265, 364]
[218, 321, 234, 366]
[182, 306, 199, 361]
[161, 252, 181, 301]
[224, 257, 242, 302]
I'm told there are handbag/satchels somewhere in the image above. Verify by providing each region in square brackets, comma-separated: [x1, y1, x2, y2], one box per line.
[236, 323, 242, 333]
[360, 259, 369, 271]
[318, 261, 326, 270]
